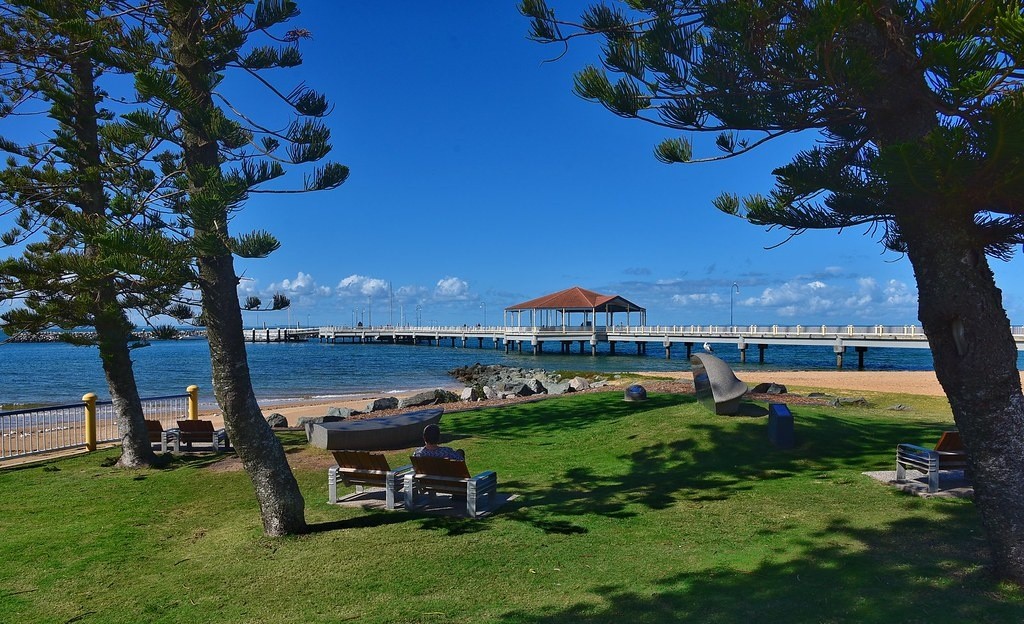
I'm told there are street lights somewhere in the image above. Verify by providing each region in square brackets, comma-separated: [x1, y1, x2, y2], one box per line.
[416, 305, 423, 329]
[729, 283, 741, 331]
[479, 301, 487, 329]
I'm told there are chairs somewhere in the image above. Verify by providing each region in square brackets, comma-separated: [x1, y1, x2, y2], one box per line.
[145, 420, 175, 453]
[403, 456, 497, 515]
[174, 420, 229, 452]
[896, 430, 969, 492]
[327, 448, 407, 509]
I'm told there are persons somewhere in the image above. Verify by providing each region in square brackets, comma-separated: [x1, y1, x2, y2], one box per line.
[411, 424, 465, 461]
